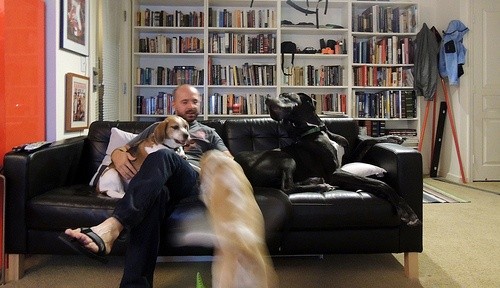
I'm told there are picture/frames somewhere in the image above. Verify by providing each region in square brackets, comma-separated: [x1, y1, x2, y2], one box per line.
[65, 73, 90, 131]
[59, 0, 90, 57]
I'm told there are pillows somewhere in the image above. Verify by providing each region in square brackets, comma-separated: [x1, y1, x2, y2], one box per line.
[89, 127, 139, 185]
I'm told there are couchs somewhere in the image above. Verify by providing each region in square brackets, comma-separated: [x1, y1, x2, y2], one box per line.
[3, 118, 423, 281]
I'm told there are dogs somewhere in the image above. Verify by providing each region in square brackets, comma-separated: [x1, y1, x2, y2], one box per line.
[198, 147, 282, 288]
[94, 115, 192, 199]
[265, 92, 348, 193]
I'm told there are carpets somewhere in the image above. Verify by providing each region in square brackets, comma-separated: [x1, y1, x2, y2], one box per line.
[422, 181, 471, 204]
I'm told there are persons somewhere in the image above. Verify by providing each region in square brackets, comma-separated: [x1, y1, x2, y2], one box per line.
[59, 83, 234, 288]
[73, 92, 85, 121]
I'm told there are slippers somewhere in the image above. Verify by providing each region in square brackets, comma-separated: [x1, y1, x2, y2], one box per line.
[57, 226, 109, 265]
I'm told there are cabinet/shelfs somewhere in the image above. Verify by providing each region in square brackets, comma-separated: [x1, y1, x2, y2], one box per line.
[131, 0, 421, 146]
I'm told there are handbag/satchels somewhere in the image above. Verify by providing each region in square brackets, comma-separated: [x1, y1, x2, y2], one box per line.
[281, 41, 297, 76]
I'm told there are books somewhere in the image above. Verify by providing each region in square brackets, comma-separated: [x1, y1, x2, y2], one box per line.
[136, 5, 420, 152]
